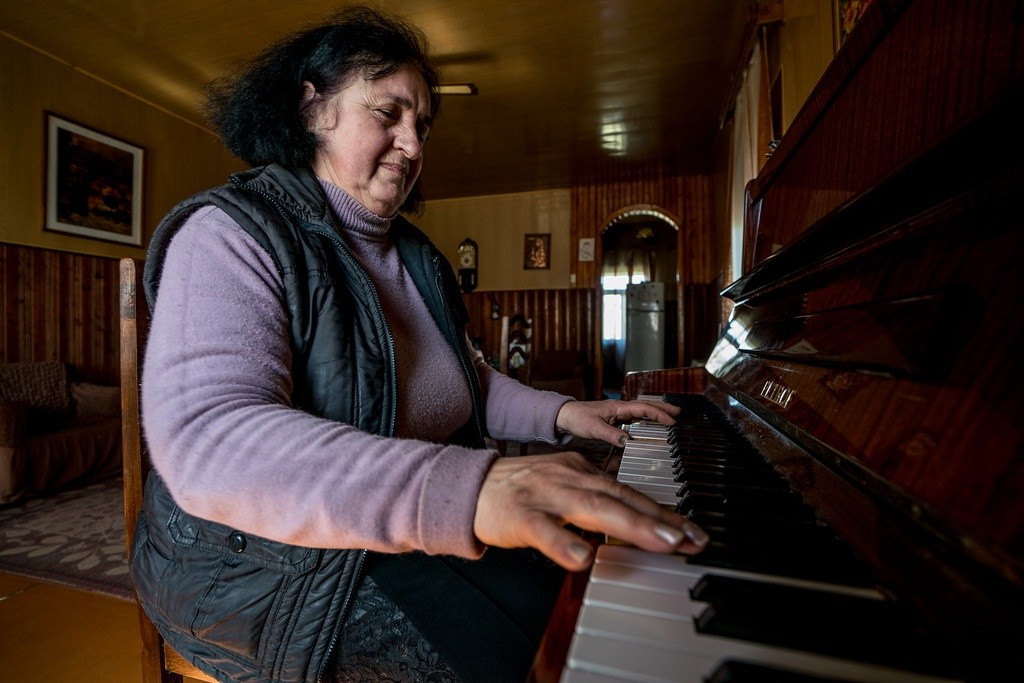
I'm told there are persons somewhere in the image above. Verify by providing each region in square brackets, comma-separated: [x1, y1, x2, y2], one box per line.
[530, 236, 546, 268]
[124, 5, 713, 683]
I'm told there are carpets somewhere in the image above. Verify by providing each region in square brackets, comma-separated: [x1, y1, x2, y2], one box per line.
[0, 472, 142, 605]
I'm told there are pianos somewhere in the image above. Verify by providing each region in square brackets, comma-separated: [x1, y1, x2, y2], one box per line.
[519, 0, 1024, 683]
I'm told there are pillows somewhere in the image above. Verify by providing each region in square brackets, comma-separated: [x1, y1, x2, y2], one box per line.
[0, 361, 72, 422]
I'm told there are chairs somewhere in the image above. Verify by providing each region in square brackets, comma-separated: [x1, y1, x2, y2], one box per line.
[118, 256, 230, 683]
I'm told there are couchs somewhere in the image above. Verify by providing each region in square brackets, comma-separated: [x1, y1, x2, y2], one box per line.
[0, 361, 149, 498]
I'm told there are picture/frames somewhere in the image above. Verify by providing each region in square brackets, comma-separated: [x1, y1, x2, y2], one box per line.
[524, 233, 552, 271]
[576, 235, 598, 264]
[42, 111, 146, 250]
